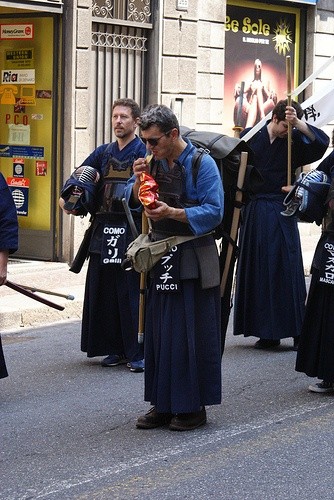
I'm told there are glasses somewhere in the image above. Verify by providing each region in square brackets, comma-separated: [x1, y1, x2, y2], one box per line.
[138, 131, 173, 146]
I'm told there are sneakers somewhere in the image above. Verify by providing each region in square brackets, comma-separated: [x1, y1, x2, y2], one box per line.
[100, 354, 144, 373]
[253, 338, 300, 351]
[307, 379, 334, 392]
[136, 405, 206, 430]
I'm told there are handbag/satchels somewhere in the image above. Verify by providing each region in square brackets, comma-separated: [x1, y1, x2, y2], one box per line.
[126, 230, 217, 273]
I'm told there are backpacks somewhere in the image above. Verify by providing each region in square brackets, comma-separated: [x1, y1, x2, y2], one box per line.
[150, 125, 250, 240]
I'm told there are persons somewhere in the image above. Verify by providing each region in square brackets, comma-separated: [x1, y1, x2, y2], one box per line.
[233, 57, 277, 134]
[281, 127, 334, 395]
[232, 98, 330, 353]
[0, 171, 19, 380]
[57, 97, 154, 374]
[123, 103, 225, 433]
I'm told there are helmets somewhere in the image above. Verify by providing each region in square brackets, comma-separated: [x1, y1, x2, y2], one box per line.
[58, 165, 101, 217]
[280, 167, 332, 223]
